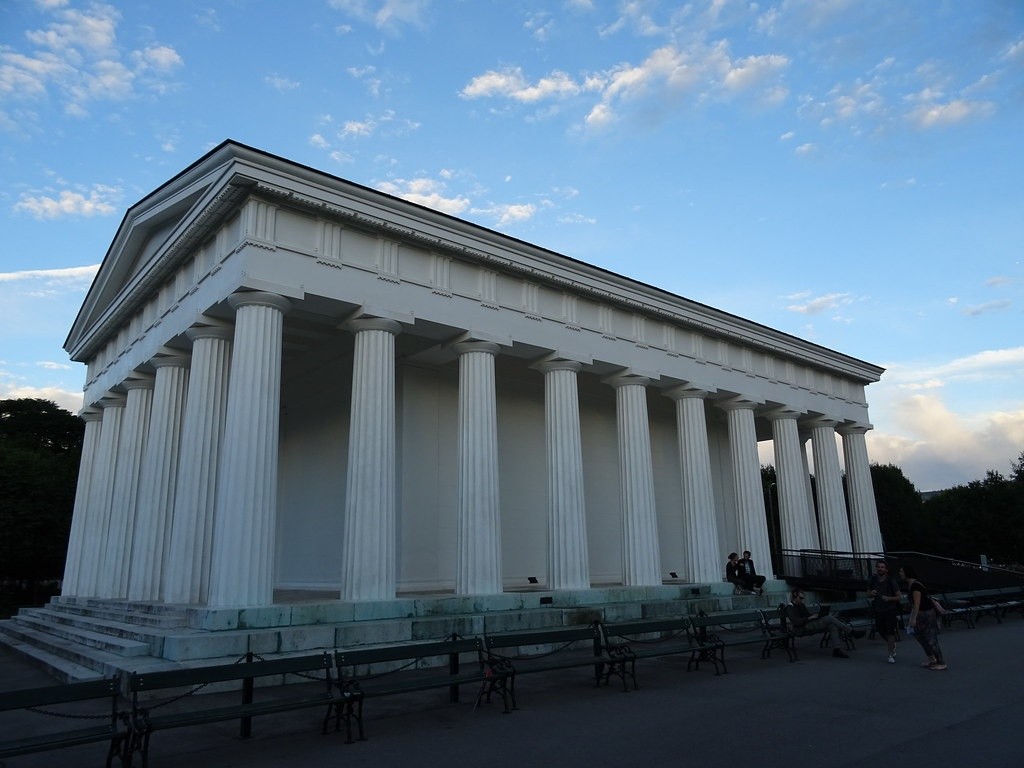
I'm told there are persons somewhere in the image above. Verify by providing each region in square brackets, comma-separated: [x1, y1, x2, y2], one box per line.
[869, 560, 902, 663]
[726, 549, 766, 597]
[786, 590, 866, 659]
[899, 564, 948, 670]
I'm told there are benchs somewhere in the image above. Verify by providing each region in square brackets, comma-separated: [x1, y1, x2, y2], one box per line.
[335, 635, 510, 744]
[897, 594, 970, 636]
[129, 651, 355, 768]
[484, 628, 627, 710]
[600, 618, 722, 690]
[819, 600, 897, 650]
[759, 606, 850, 662]
[1000, 586, 1024, 619]
[868, 597, 940, 641]
[0, 674, 134, 768]
[973, 589, 1013, 625]
[689, 612, 794, 676]
[943, 592, 996, 631]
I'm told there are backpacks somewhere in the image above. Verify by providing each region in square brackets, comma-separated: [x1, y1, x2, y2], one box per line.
[928, 594, 949, 615]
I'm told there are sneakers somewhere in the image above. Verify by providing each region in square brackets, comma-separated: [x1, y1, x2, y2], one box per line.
[888, 655, 896, 664]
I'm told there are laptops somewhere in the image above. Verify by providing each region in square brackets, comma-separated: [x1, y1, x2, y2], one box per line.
[809, 605, 831, 621]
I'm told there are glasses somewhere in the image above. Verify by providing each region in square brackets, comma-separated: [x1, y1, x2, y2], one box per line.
[796, 593, 805, 600]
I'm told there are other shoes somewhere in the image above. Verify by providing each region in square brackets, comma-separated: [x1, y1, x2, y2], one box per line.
[848, 629, 867, 640]
[920, 657, 948, 670]
[833, 648, 849, 659]
